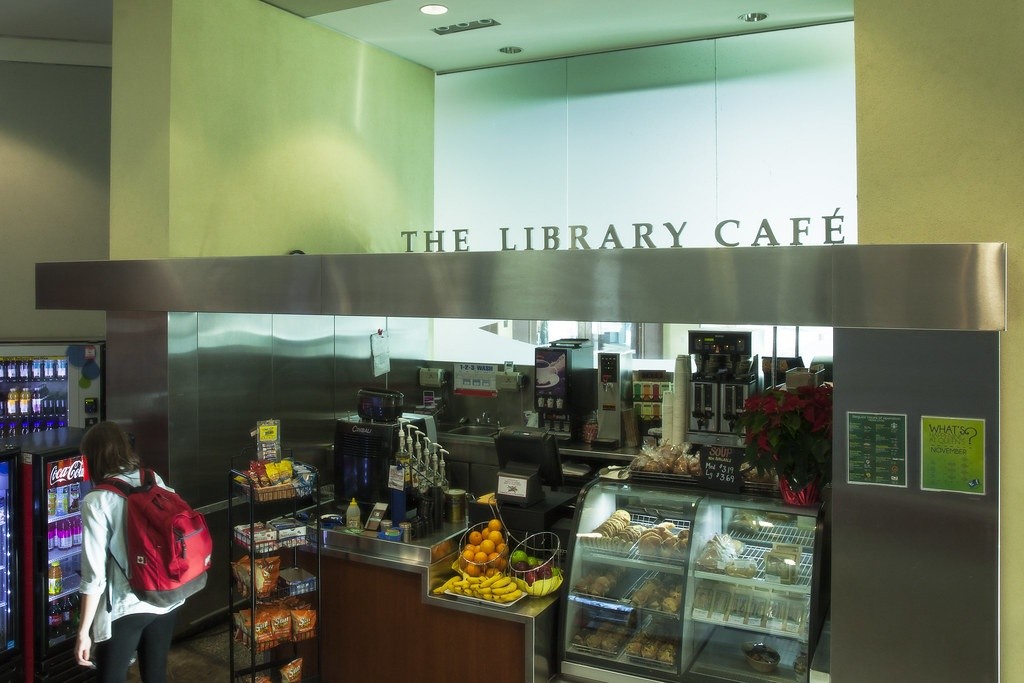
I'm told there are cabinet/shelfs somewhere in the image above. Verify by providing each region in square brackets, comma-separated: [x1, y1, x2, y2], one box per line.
[556, 475, 829, 683]
[228, 462, 324, 683]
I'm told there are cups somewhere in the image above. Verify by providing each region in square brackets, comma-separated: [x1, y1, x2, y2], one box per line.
[544, 421, 570, 432]
[662, 354, 691, 446]
[538, 399, 564, 408]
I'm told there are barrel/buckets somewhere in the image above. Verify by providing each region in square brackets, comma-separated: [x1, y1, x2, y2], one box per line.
[444, 488, 466, 524]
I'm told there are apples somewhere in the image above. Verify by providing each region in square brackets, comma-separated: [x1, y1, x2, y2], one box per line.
[511, 551, 552, 586]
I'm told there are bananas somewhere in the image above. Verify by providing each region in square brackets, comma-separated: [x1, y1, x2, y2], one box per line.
[432, 571, 522, 603]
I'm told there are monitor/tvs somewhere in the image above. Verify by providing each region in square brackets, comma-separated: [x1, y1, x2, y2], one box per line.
[494, 431, 564, 487]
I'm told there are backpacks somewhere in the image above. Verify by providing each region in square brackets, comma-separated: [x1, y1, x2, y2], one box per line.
[91, 467, 213, 613]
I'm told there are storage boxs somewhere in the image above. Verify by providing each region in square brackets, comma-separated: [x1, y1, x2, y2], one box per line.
[234, 518, 306, 552]
[786, 367, 825, 389]
[278, 566, 316, 596]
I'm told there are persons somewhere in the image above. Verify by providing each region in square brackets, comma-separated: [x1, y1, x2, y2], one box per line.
[74, 421, 184, 683]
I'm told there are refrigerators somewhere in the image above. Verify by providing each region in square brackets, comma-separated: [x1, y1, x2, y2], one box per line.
[0, 426, 134, 683]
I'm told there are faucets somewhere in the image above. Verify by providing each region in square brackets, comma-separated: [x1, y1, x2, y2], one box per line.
[474, 409, 491, 424]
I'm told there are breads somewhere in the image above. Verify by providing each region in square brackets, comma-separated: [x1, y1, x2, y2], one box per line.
[576, 508, 690, 663]
[630, 449, 700, 475]
[696, 532, 758, 580]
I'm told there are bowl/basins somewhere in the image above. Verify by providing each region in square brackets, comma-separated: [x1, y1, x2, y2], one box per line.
[741, 642, 781, 672]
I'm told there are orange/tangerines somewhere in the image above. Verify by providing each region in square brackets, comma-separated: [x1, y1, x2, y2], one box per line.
[461, 518, 509, 576]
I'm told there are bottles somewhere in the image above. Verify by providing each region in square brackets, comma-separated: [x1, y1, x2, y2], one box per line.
[0, 384, 67, 439]
[346, 498, 360, 528]
[399, 522, 411, 544]
[48, 482, 82, 640]
[380, 519, 393, 532]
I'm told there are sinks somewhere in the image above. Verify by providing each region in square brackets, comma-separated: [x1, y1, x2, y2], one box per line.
[436, 425, 504, 437]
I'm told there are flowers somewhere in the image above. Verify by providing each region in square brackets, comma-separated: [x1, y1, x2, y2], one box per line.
[727, 385, 833, 493]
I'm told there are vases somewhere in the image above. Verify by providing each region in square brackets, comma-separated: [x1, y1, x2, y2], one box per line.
[778, 474, 823, 505]
